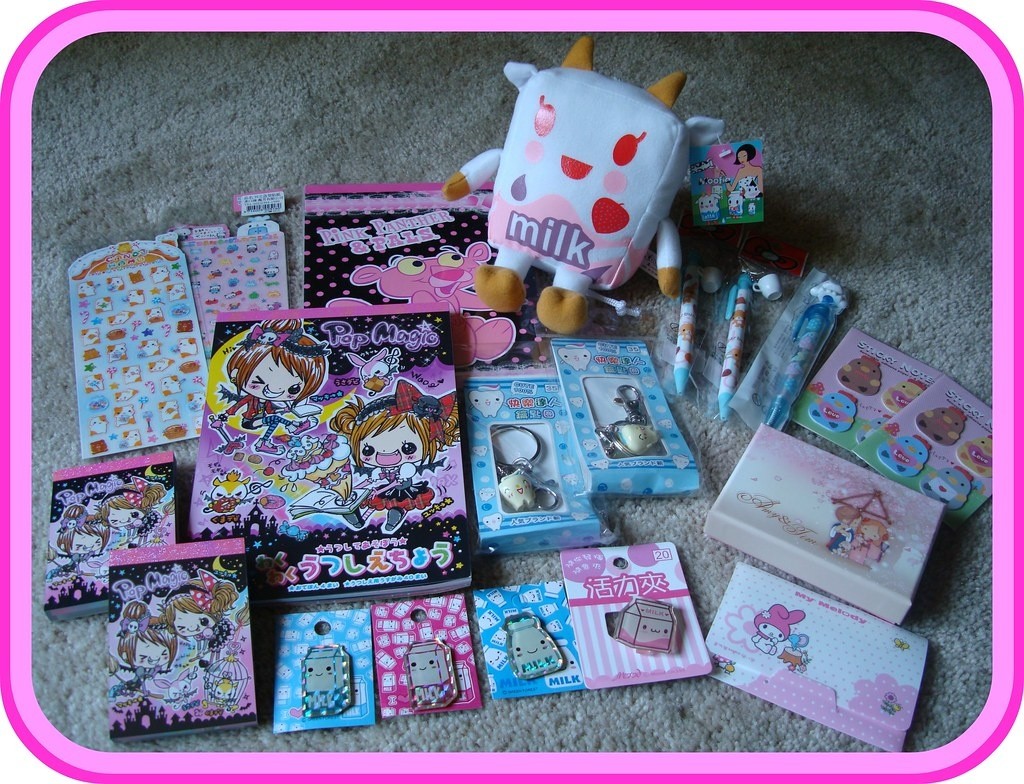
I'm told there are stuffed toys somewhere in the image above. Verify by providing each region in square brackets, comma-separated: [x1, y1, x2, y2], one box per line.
[440, 36, 724, 334]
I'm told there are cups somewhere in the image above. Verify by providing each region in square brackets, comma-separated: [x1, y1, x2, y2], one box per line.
[698, 267, 722, 294]
[753, 273, 782, 301]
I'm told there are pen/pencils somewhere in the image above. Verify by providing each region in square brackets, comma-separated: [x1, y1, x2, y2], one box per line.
[716, 274, 752, 421]
[674, 267, 700, 394]
[765, 296, 833, 427]
